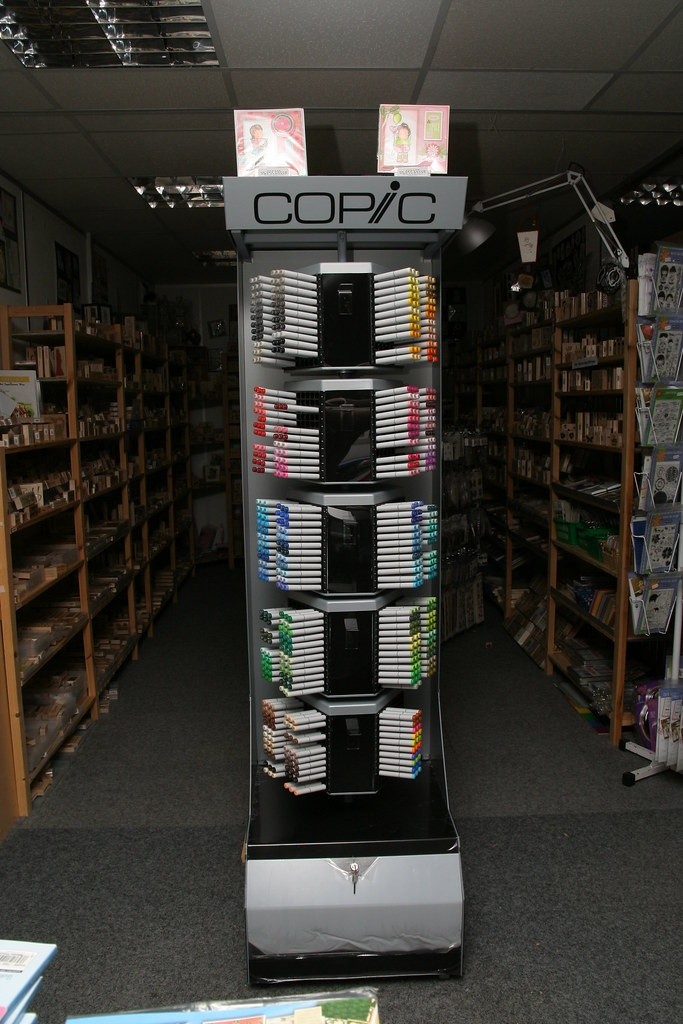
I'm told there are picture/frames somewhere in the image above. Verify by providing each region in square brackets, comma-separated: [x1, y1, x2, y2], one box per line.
[207, 348, 222, 372]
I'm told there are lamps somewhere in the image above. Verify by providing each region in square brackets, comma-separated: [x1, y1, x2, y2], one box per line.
[454, 168, 630, 270]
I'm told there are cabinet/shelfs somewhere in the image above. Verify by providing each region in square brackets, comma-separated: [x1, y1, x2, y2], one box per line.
[220, 352, 243, 570]
[451, 277, 683, 747]
[0, 303, 196, 818]
[222, 174, 472, 989]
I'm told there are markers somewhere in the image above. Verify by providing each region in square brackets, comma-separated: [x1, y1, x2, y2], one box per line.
[250, 268, 327, 796]
[373, 267, 439, 780]
